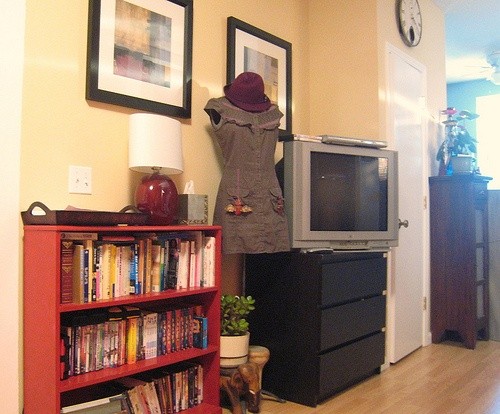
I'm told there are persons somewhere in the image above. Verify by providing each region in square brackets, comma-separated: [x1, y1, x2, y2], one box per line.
[203, 72, 291, 253]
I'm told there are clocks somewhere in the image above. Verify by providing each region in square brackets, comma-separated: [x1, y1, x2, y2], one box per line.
[395, 0, 422, 46]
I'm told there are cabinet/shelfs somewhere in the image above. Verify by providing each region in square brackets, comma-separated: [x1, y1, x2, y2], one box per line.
[246, 249, 391, 408]
[422, 175, 493, 351]
[22, 224, 224, 414]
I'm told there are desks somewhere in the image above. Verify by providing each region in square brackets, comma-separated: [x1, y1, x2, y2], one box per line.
[220, 345, 271, 414]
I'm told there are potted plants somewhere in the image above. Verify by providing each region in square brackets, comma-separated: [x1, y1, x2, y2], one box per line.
[219, 295, 258, 368]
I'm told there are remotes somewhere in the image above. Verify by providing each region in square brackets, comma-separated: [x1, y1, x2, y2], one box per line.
[306, 248, 333, 253]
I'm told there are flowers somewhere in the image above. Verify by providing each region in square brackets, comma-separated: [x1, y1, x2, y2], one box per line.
[433, 107, 480, 157]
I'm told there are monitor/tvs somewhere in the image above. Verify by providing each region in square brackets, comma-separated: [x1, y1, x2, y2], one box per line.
[274, 140, 400, 250]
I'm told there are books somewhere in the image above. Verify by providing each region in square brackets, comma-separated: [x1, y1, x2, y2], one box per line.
[60, 231, 215, 303]
[60, 301, 208, 381]
[60, 363, 204, 414]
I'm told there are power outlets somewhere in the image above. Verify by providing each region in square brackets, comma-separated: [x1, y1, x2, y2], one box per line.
[69, 166, 94, 194]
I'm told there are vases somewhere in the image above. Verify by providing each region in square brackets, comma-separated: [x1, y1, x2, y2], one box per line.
[448, 153, 475, 173]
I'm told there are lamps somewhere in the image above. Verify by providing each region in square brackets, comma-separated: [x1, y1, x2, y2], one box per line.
[125, 113, 183, 224]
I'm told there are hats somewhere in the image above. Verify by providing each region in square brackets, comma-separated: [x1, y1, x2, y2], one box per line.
[224, 72, 271, 112]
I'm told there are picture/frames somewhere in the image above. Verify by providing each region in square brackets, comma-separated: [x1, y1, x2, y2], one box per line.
[225, 16, 292, 140]
[86, 0, 194, 119]
